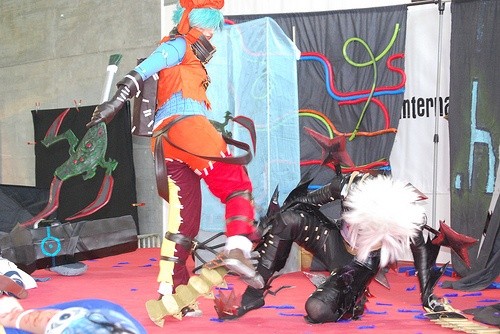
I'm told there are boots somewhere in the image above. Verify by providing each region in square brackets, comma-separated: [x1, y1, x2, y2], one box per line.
[224, 237, 264, 289]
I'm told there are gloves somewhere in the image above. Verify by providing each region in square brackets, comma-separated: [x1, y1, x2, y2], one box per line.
[424, 299, 458, 317]
[88, 71, 144, 128]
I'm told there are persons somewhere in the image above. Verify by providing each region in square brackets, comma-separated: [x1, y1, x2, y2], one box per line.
[84, 0, 265, 317]
[226, 169, 447, 325]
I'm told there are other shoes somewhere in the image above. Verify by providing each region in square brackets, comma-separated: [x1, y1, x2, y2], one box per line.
[220, 294, 266, 319]
